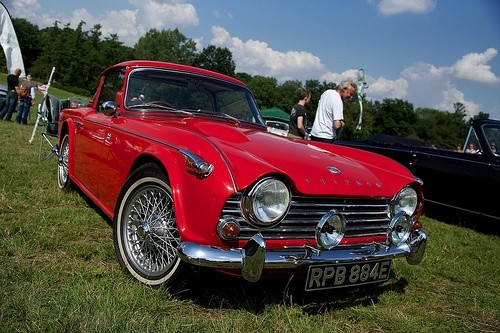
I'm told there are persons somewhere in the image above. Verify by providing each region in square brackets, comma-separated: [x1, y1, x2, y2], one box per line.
[15, 74, 35, 125]
[311, 80, 357, 144]
[453, 143, 500, 157]
[0, 69, 22, 121]
[287, 89, 311, 140]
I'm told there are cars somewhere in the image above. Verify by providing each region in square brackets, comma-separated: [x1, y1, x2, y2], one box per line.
[56, 60, 428, 294]
[333, 118, 500, 229]
